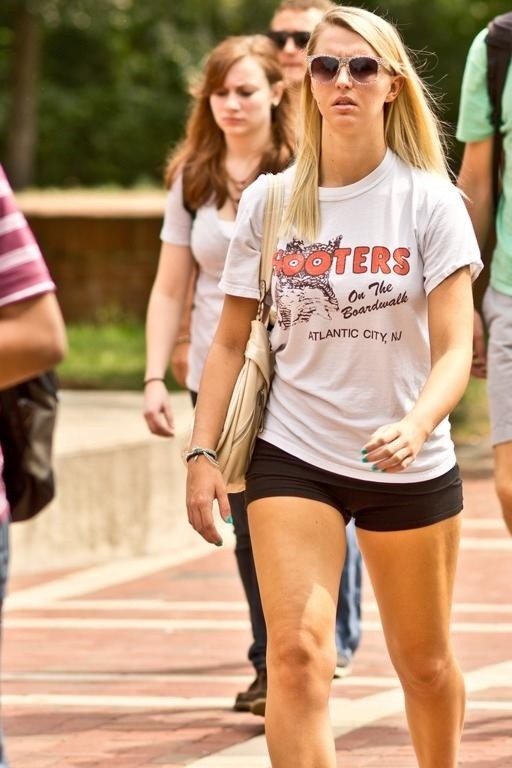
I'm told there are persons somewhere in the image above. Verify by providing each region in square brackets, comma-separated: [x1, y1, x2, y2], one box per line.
[186, 7, 483, 768]
[450, 9, 510, 531]
[143, 36, 274, 714]
[267, 0, 362, 680]
[0, 162, 71, 767]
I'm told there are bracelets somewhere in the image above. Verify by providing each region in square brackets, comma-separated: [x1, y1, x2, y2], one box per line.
[143, 377, 164, 386]
[182, 447, 219, 463]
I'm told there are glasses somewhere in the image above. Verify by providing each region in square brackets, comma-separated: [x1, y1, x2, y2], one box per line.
[306, 55, 392, 84]
[267, 31, 311, 50]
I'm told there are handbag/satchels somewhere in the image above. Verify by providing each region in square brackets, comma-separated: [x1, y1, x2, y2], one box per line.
[0, 369, 57, 523]
[212, 320, 273, 495]
[171, 264, 197, 386]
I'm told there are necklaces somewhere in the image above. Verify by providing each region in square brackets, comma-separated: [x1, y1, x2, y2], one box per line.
[224, 165, 260, 216]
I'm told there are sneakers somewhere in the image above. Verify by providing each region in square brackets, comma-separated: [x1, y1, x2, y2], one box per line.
[234, 668, 266, 716]
[334, 654, 352, 678]
